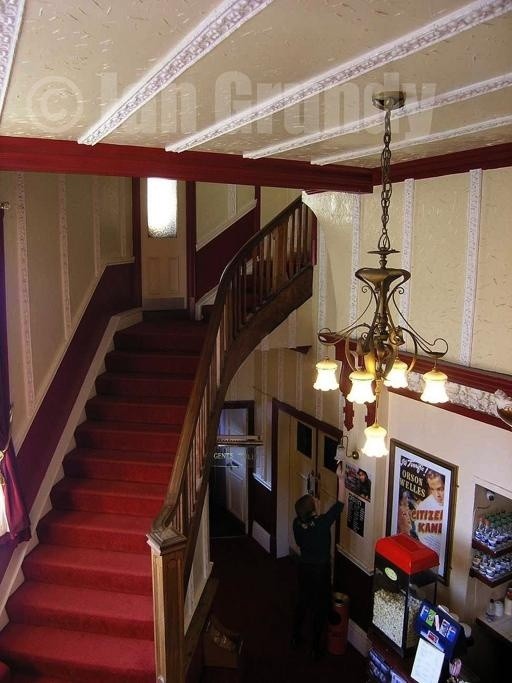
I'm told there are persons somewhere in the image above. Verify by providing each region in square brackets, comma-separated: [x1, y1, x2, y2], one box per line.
[399, 470, 445, 539]
[355, 470, 371, 498]
[288, 464, 346, 664]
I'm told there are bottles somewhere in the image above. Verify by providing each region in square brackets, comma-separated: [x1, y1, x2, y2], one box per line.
[485, 587, 512, 621]
[470, 509, 512, 583]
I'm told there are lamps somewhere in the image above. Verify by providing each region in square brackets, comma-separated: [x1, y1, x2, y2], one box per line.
[312, 87, 451, 459]
[333, 435, 359, 462]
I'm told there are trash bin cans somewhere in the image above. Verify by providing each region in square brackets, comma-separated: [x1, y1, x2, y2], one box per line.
[326, 592, 350, 657]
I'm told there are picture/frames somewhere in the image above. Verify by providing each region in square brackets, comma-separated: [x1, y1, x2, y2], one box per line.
[385, 436, 458, 586]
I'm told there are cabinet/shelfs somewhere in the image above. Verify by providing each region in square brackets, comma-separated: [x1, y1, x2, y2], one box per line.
[469, 539, 511, 589]
